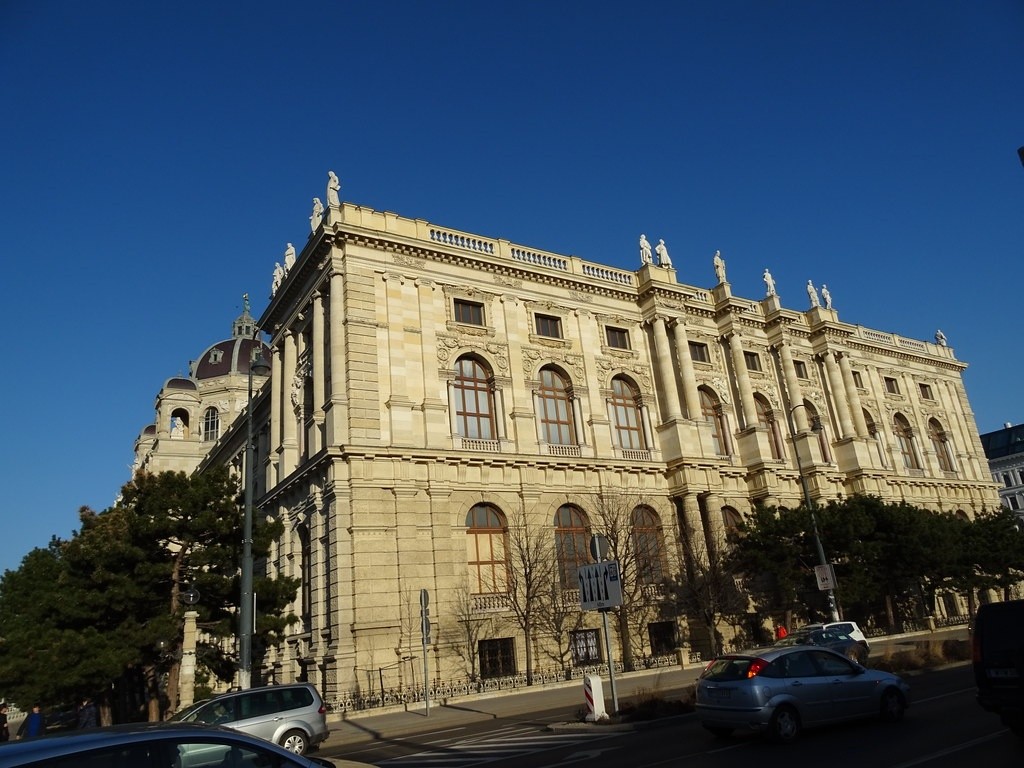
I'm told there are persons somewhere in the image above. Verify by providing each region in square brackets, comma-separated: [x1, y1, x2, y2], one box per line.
[822, 285, 833, 310]
[655, 239, 674, 269]
[201, 705, 230, 725]
[713, 251, 725, 285]
[763, 269, 776, 297]
[806, 279, 821, 308]
[14, 704, 48, 740]
[272, 170, 341, 299]
[934, 330, 947, 346]
[640, 234, 653, 266]
[0, 703, 10, 744]
[773, 623, 788, 643]
[76, 697, 96, 730]
[175, 417, 185, 432]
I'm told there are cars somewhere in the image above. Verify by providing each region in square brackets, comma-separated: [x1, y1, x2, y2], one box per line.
[770, 621, 871, 668]
[694, 645, 914, 748]
[0, 720, 382, 768]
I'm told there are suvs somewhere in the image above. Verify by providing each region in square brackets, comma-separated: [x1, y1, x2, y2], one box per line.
[970, 599, 1024, 737]
[166, 681, 330, 768]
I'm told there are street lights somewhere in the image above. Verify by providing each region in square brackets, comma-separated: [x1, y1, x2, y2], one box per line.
[238, 329, 273, 695]
[787, 404, 841, 623]
